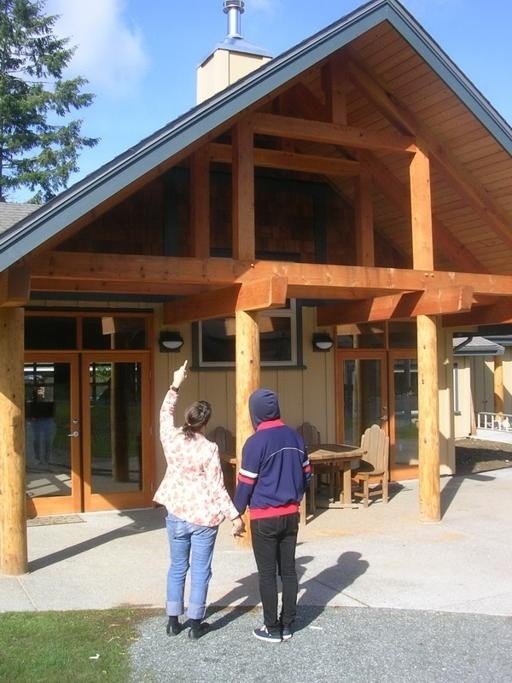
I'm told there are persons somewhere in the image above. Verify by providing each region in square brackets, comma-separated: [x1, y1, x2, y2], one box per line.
[29, 384, 57, 466]
[152, 359, 245, 639]
[230, 387, 313, 642]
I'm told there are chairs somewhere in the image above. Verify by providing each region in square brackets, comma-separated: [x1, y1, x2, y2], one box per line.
[207, 423, 392, 543]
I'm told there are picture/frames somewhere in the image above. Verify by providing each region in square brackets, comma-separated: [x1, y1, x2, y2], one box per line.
[193, 247, 304, 371]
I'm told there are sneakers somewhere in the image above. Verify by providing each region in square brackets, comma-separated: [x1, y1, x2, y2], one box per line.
[189, 622, 210, 638]
[167, 623, 184, 636]
[281, 628, 293, 640]
[252, 627, 282, 642]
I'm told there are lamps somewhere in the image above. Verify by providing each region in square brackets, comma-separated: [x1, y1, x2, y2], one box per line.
[311, 331, 333, 353]
[157, 332, 185, 355]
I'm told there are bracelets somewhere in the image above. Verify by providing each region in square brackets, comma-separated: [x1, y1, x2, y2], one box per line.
[170, 383, 180, 392]
